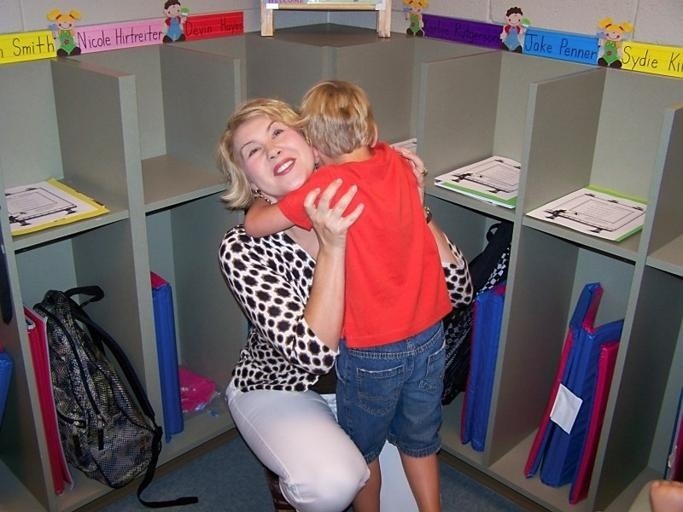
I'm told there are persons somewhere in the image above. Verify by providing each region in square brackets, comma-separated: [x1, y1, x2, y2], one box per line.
[217, 98, 473, 511]
[245, 81, 453, 511]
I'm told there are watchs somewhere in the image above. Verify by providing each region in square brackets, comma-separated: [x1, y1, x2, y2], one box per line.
[423, 205, 433, 223]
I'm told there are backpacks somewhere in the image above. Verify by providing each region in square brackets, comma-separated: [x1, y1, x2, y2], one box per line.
[33, 285, 199, 508]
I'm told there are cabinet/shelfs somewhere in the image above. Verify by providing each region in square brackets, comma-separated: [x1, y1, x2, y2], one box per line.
[333, 26, 683, 511]
[0, 24, 329, 511]
